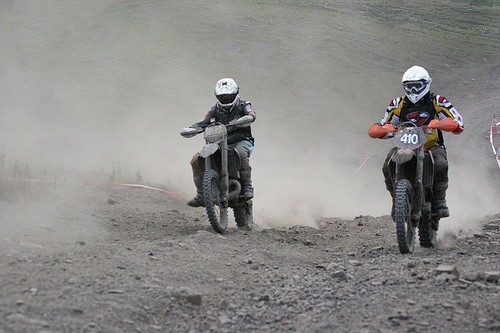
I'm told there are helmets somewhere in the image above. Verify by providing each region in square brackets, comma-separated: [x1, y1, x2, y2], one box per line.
[401, 65, 431, 103]
[214, 78, 240, 113]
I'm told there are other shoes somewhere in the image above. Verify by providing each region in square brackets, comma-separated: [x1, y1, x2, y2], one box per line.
[391, 199, 398, 221]
[437, 208, 450, 217]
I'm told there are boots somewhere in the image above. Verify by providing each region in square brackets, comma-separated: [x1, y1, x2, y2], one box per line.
[239, 167, 253, 198]
[187, 178, 203, 208]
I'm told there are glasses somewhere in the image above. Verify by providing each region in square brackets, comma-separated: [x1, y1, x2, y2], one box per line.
[404, 81, 425, 93]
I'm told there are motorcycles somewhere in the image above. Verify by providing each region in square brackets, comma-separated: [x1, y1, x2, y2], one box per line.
[180, 114, 256, 235]
[369, 117, 459, 255]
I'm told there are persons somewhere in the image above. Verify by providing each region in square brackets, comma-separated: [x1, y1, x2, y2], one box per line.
[369, 66, 463, 221]
[181, 78, 255, 207]
